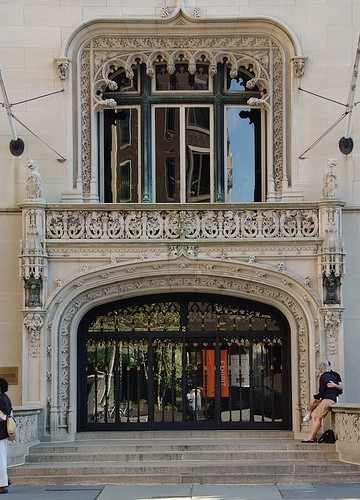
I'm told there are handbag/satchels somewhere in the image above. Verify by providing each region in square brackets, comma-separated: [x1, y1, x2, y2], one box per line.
[7, 409, 16, 442]
[318, 429, 335, 444]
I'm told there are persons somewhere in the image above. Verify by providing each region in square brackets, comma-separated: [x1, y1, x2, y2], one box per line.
[300, 361, 344, 443]
[0, 377, 14, 495]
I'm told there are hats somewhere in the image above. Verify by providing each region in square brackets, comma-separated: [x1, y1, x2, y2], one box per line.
[320, 361, 329, 372]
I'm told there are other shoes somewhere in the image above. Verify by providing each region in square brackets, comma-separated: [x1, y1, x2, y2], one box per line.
[304, 412, 312, 422]
[0, 487, 8, 494]
[8, 478, 12, 486]
[301, 437, 316, 443]
